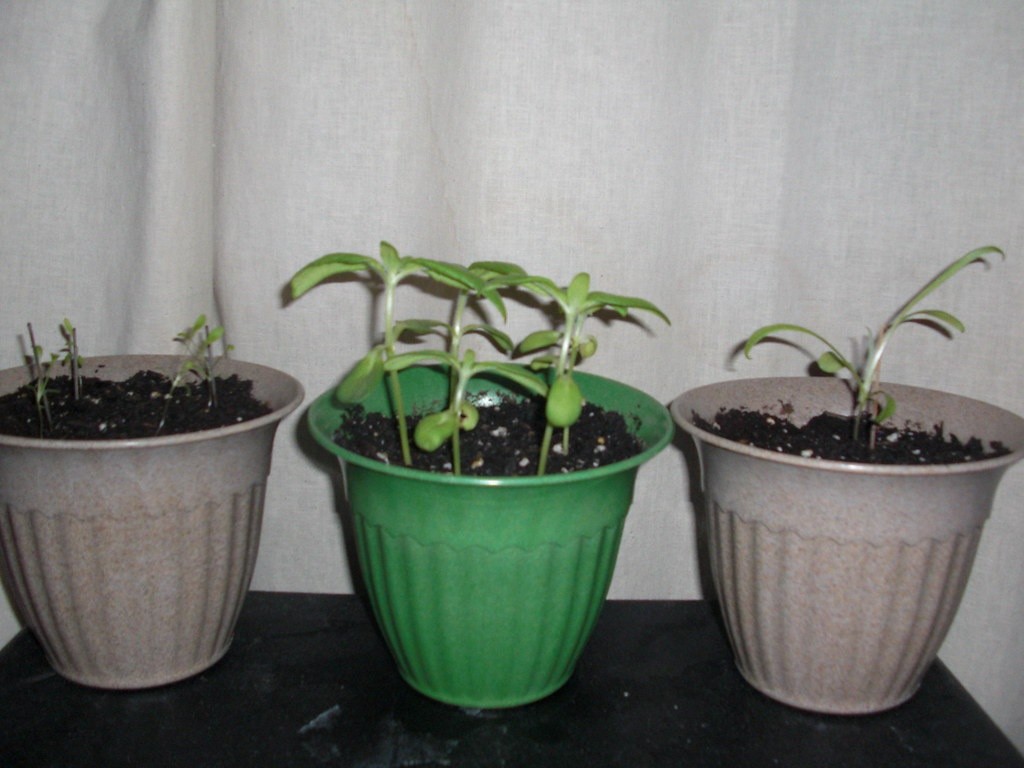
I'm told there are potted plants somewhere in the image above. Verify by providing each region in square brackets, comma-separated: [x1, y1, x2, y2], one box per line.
[0, 311, 305, 687]
[289, 246, 674, 708]
[670, 243, 1024, 715]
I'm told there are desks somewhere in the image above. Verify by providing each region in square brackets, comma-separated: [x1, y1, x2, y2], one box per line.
[0, 592, 1024, 768]
